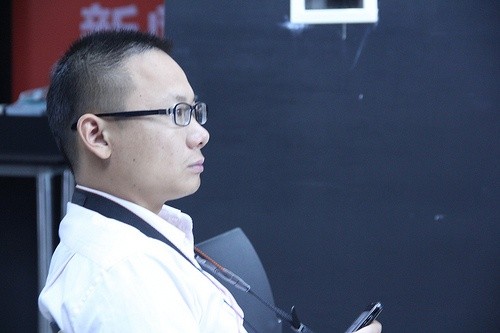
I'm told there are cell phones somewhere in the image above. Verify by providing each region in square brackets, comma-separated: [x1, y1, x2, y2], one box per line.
[344, 301, 384, 333]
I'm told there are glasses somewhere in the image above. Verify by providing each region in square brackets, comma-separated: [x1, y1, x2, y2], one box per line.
[72, 101, 208, 131]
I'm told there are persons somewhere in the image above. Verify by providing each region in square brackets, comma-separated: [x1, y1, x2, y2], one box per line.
[37, 28, 383, 333]
